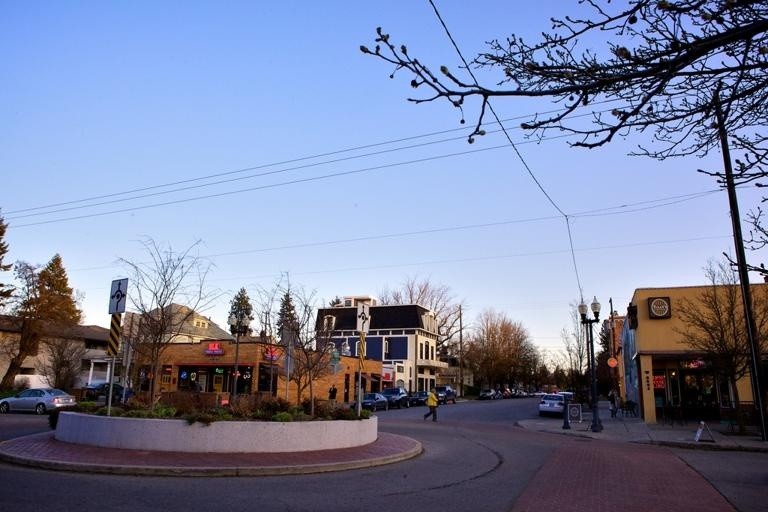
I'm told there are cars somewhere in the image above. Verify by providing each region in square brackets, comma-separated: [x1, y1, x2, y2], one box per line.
[539, 390, 576, 415]
[478, 388, 545, 400]
[362, 386, 457, 411]
[0, 388, 76, 415]
[81, 383, 124, 403]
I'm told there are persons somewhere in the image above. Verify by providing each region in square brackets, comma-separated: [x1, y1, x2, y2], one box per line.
[607, 388, 617, 418]
[423, 387, 439, 424]
[327, 384, 338, 405]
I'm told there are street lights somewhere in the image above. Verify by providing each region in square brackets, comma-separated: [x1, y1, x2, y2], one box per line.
[578, 296, 603, 427]
[229, 314, 249, 396]
